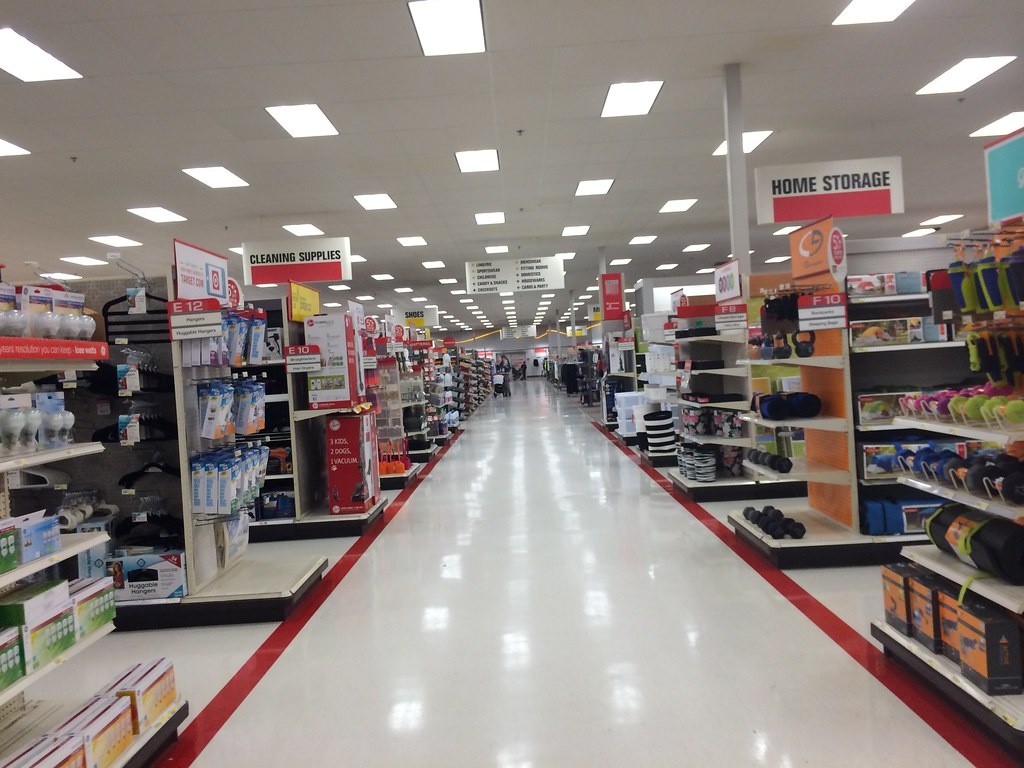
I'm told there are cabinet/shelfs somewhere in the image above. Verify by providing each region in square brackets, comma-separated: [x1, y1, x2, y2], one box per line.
[600, 315, 649, 447]
[650, 271, 808, 503]
[868, 124, 1024, 763]
[0, 286, 193, 768]
[65, 262, 331, 633]
[242, 291, 495, 539]
[726, 216, 984, 570]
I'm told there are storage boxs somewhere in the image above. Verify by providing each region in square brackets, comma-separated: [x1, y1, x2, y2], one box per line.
[325, 411, 380, 515]
[880, 559, 1024, 695]
[0, 509, 188, 768]
[842, 270, 995, 535]
[303, 312, 368, 408]
[613, 295, 808, 458]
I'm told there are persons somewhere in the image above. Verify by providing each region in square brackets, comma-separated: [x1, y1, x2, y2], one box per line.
[500, 354, 511, 397]
[521, 361, 527, 381]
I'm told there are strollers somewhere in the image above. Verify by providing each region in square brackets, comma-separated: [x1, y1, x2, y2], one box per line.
[493, 373, 507, 397]
